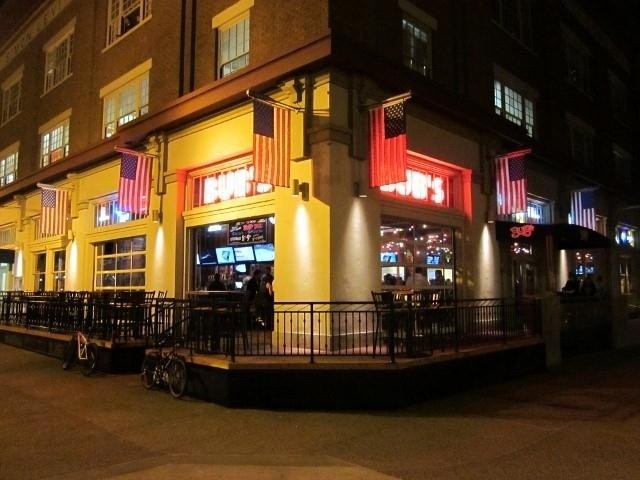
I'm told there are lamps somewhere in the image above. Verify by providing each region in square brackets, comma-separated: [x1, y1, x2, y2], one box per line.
[292, 179, 309, 201]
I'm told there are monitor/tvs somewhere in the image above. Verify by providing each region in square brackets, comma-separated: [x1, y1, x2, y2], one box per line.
[426, 255, 440, 264]
[201, 241, 274, 266]
[381, 253, 398, 263]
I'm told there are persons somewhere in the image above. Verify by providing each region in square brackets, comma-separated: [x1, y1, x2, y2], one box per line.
[115, 16, 133, 37]
[208, 265, 275, 330]
[563, 270, 608, 294]
[379, 266, 454, 330]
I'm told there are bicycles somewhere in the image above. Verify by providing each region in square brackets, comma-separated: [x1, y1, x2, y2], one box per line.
[61, 329, 98, 376]
[141, 342, 188, 398]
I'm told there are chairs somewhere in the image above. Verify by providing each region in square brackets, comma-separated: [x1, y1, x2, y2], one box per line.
[371, 289, 460, 359]
[0, 290, 249, 361]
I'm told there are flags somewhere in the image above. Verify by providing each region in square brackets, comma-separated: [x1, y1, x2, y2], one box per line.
[118, 149, 153, 216]
[251, 93, 291, 189]
[494, 152, 529, 215]
[367, 96, 408, 188]
[569, 188, 596, 232]
[40, 184, 68, 235]
